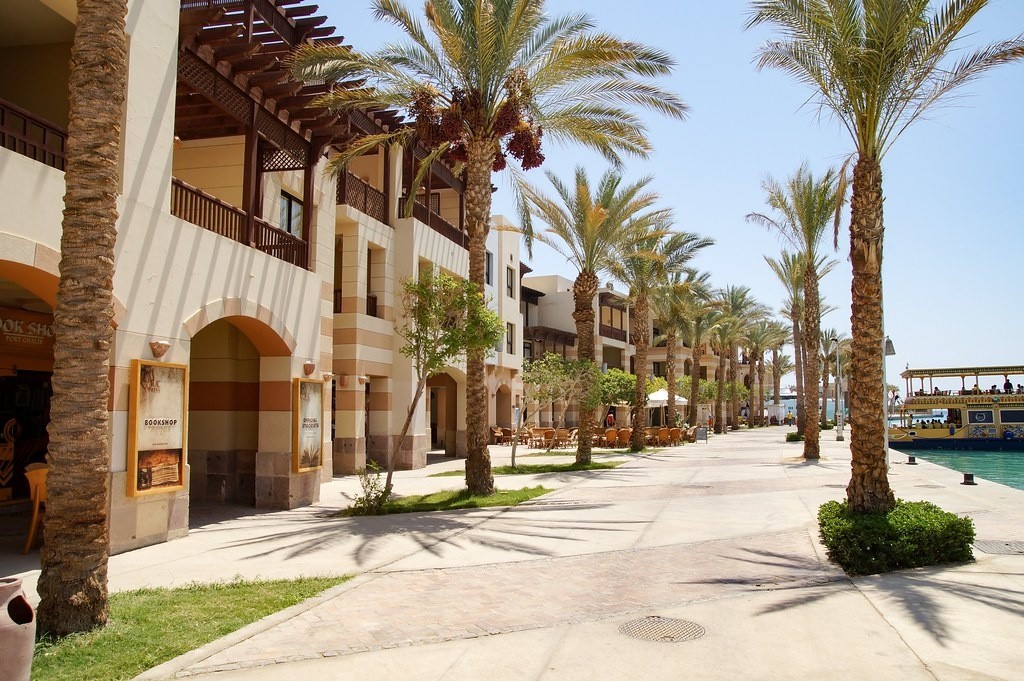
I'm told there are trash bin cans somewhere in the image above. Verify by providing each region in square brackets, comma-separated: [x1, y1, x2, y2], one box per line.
[754, 416, 760, 424]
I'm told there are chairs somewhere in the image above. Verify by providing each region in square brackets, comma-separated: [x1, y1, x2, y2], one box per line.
[489, 426, 685, 450]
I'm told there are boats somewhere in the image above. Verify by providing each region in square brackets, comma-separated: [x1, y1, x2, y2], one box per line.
[888, 362, 1024, 451]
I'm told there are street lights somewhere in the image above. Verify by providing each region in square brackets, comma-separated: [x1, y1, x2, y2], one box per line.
[882, 334, 896, 468]
[829, 337, 845, 442]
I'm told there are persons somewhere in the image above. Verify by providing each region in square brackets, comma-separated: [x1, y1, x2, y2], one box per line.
[934, 387, 940, 396]
[706, 416, 712, 432]
[916, 416, 961, 429]
[920, 389, 924, 396]
[992, 385, 997, 394]
[1004, 379, 1013, 394]
[795, 414, 798, 427]
[607, 410, 614, 428]
[1016, 384, 1024, 394]
[788, 411, 793, 426]
[907, 414, 913, 429]
[972, 384, 980, 395]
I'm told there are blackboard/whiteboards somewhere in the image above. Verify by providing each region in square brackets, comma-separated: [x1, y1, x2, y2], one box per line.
[695, 427, 707, 440]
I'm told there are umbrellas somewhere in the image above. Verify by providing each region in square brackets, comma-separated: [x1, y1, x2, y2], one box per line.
[643, 388, 688, 427]
